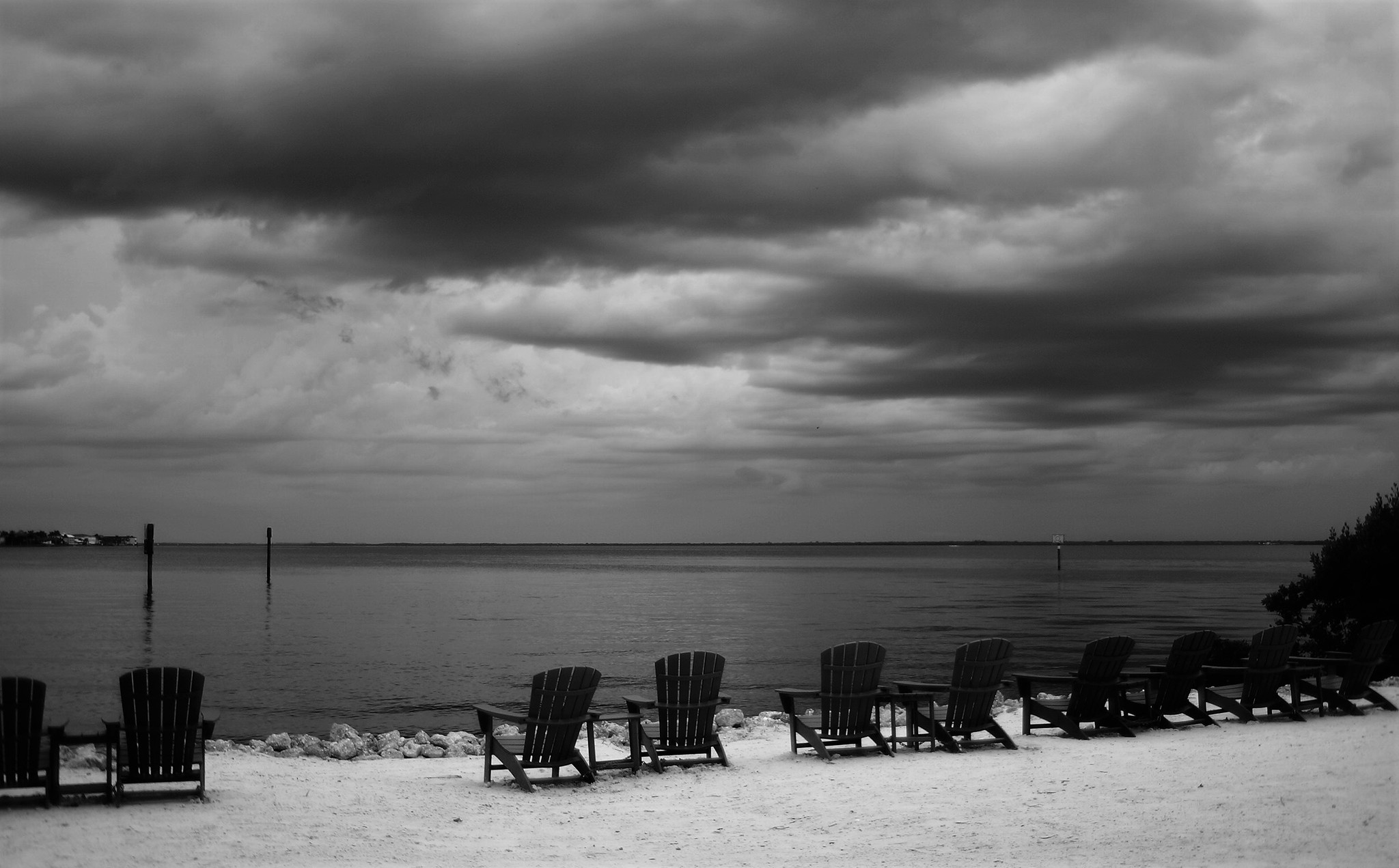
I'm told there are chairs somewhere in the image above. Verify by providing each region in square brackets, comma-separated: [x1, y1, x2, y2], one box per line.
[0, 675, 68, 802]
[1197, 624, 1311, 724]
[775, 641, 895, 763]
[622, 650, 733, 774]
[1013, 636, 1139, 740]
[892, 637, 1018, 755]
[1119, 629, 1222, 730]
[470, 664, 603, 794]
[101, 665, 216, 807]
[1286, 619, 1398, 716]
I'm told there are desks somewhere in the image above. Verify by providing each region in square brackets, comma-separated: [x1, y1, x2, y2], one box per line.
[60, 721, 112, 803]
[874, 690, 938, 753]
[1115, 676, 1154, 729]
[1289, 665, 1325, 717]
[589, 713, 644, 774]
